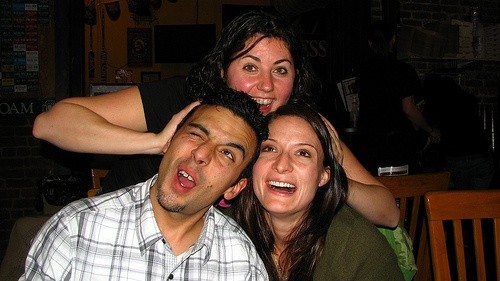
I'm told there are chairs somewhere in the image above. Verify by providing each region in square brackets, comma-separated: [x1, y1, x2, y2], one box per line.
[375, 172, 500, 281]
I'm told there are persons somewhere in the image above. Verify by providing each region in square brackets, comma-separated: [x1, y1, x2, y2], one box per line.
[234, 103, 404, 281]
[357, 20, 492, 191]
[32, 10, 399, 229]
[132, 36, 149, 67]
[17, 89, 269, 281]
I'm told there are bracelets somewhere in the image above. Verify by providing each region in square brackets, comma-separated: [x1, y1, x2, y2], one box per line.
[426, 128, 433, 133]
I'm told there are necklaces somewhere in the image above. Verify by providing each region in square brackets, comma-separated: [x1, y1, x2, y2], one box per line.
[272, 253, 288, 280]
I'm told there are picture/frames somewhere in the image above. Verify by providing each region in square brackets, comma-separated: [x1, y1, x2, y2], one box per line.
[141, 71, 161, 85]
